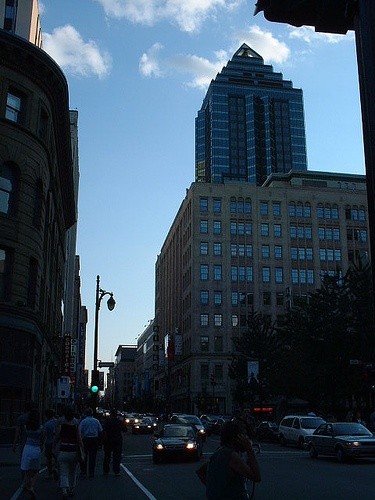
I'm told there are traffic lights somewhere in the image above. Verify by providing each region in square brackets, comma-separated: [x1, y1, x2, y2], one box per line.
[91, 370, 99, 395]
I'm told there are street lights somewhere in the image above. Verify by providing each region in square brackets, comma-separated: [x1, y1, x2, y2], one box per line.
[93, 275, 116, 371]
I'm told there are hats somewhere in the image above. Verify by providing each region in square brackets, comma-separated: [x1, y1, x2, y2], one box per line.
[221, 423, 244, 434]
[85, 407, 93, 415]
[109, 408, 116, 413]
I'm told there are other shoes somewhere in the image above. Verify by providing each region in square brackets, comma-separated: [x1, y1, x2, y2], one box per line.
[28, 489, 37, 500]
[115, 473, 120, 476]
[88, 473, 94, 477]
[69, 489, 74, 495]
[63, 489, 67, 495]
[103, 472, 108, 476]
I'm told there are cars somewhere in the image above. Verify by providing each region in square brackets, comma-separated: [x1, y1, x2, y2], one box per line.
[152, 413, 233, 464]
[303, 422, 375, 461]
[95, 407, 160, 433]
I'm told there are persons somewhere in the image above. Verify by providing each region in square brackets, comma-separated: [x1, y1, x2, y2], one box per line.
[230, 408, 257, 457]
[43, 404, 83, 481]
[79, 407, 103, 478]
[20, 409, 46, 500]
[52, 410, 85, 500]
[102, 410, 127, 476]
[206, 420, 261, 500]
[11, 400, 33, 492]
[354, 410, 368, 425]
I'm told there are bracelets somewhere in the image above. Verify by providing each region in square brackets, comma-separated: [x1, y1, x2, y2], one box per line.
[247, 446, 256, 461]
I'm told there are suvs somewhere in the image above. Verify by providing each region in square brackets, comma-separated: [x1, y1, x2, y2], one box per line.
[277, 415, 330, 449]
